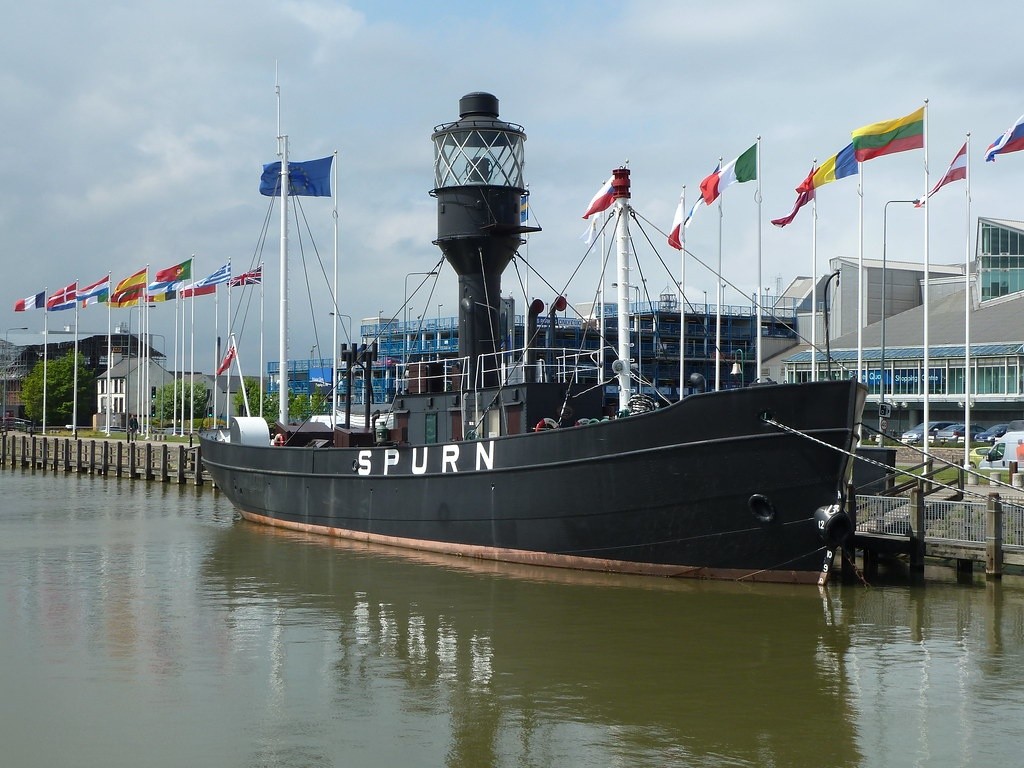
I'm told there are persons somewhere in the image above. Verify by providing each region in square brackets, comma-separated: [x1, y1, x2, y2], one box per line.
[129, 415, 138, 442]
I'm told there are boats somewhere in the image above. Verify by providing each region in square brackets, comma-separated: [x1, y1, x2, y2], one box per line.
[196, 88, 878, 589]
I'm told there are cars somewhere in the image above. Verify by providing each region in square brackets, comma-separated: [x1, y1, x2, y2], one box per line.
[164, 427, 187, 436]
[137, 424, 159, 433]
[901, 421, 960, 447]
[974, 423, 1010, 444]
[1006, 420, 1024, 433]
[927, 423, 988, 444]
[967, 446, 1005, 469]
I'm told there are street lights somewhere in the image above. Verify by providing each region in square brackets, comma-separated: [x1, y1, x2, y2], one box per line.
[125, 305, 156, 441]
[377, 309, 390, 336]
[438, 304, 444, 328]
[141, 332, 166, 429]
[328, 311, 353, 350]
[729, 348, 749, 392]
[765, 286, 770, 316]
[2, 328, 25, 424]
[721, 283, 728, 314]
[408, 306, 413, 331]
[609, 282, 644, 395]
[402, 272, 438, 393]
[876, 199, 926, 447]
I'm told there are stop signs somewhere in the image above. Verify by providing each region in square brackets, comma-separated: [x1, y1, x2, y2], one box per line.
[881, 420, 890, 430]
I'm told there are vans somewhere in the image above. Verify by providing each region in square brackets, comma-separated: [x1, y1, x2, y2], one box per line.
[977, 432, 1024, 474]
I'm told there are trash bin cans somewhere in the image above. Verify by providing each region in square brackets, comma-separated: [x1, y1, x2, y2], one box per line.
[852, 447, 897, 495]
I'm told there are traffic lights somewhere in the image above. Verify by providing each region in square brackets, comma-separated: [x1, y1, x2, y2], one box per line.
[245, 388, 249, 399]
[208, 407, 214, 418]
[150, 386, 158, 399]
[151, 404, 161, 419]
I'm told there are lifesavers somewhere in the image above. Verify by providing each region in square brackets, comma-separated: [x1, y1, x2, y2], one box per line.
[576, 418, 590, 426]
[535, 417, 558, 432]
[274, 432, 284, 446]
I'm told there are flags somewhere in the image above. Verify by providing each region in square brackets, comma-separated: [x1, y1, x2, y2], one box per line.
[47, 282, 77, 311]
[106, 269, 147, 307]
[668, 193, 684, 251]
[197, 263, 231, 288]
[699, 143, 757, 206]
[851, 107, 923, 162]
[227, 266, 262, 287]
[913, 143, 967, 207]
[14, 291, 45, 312]
[984, 114, 1024, 162]
[520, 196, 528, 222]
[180, 279, 216, 298]
[155, 259, 192, 282]
[684, 163, 720, 227]
[77, 276, 109, 308]
[145, 280, 184, 302]
[770, 166, 814, 228]
[259, 155, 334, 197]
[582, 177, 617, 252]
[796, 142, 860, 194]
